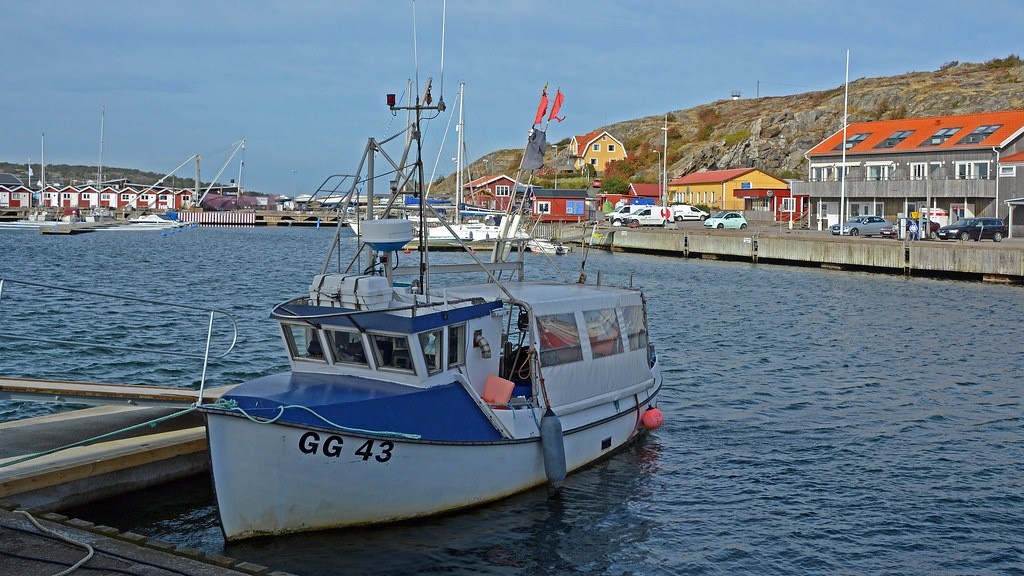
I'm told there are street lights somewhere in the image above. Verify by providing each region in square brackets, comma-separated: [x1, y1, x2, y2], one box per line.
[291, 169, 297, 207]
[652, 150, 661, 201]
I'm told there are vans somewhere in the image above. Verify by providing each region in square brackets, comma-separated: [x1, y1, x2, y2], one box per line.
[621, 206, 675, 228]
[604, 205, 656, 227]
[671, 205, 709, 222]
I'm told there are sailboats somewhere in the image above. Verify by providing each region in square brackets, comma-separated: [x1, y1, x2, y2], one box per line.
[27, 133, 54, 221]
[348, 83, 530, 249]
[85, 104, 117, 222]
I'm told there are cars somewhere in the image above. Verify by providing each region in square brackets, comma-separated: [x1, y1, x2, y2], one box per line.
[703, 211, 748, 230]
[880, 218, 940, 239]
[829, 215, 894, 237]
[936, 217, 1005, 242]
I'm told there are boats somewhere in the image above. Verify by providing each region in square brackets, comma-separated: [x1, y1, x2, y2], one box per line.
[190, 0, 665, 545]
[528, 241, 570, 255]
[59, 206, 84, 222]
